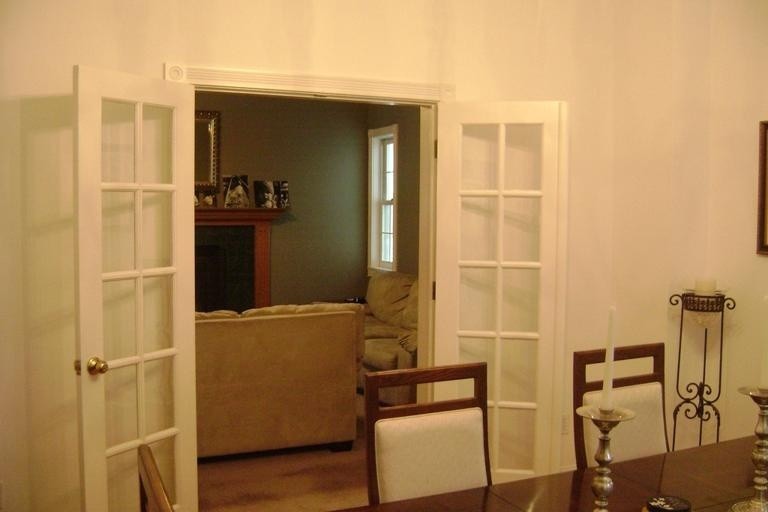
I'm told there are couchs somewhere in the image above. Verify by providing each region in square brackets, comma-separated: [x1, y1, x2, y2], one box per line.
[195, 294, 419, 460]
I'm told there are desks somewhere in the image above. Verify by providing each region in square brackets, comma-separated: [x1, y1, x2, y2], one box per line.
[331, 428, 768, 512]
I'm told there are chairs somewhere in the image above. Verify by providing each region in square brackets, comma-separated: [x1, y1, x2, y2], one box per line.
[572, 342, 669, 467]
[364, 361, 492, 504]
[138, 444, 174, 512]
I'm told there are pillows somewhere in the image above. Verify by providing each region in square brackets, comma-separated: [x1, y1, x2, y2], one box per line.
[366, 269, 419, 327]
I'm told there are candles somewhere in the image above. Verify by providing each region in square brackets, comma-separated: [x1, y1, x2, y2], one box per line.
[694, 274, 717, 296]
[760, 295, 768, 390]
[602, 303, 615, 412]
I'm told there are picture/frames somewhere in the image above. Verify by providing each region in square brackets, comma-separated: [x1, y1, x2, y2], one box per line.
[756, 120, 768, 254]
[195, 110, 220, 193]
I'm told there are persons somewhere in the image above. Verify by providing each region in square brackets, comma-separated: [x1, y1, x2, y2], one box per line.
[280, 182, 290, 208]
[255, 181, 279, 208]
